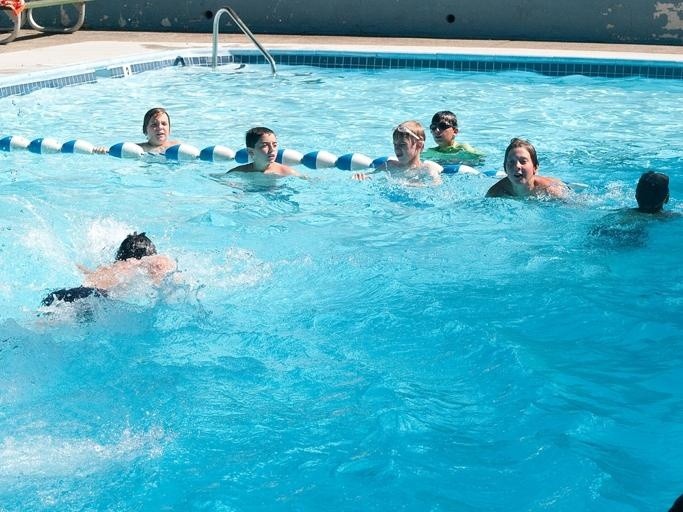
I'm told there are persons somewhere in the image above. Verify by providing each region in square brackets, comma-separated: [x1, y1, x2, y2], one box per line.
[346, 117, 445, 189]
[613, 169, 683, 220]
[91, 105, 182, 157]
[486, 134, 570, 207]
[34, 223, 177, 316]
[428, 107, 474, 156]
[223, 125, 315, 184]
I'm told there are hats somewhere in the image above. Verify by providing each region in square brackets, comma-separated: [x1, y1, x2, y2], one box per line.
[636, 172, 668, 205]
[116, 235, 157, 260]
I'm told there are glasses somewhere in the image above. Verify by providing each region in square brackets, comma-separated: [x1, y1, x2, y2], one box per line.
[430, 122, 455, 130]
[392, 125, 420, 140]
